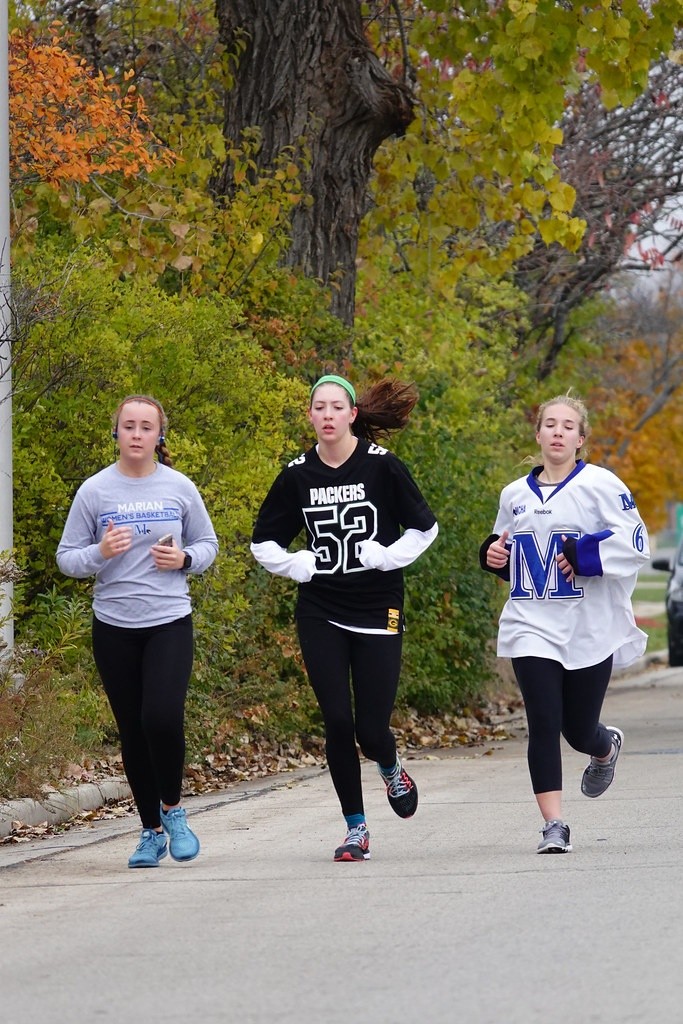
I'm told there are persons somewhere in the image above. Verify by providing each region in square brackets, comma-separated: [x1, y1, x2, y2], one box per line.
[478, 395, 651, 855]
[56, 393, 219, 867]
[252, 377, 440, 864]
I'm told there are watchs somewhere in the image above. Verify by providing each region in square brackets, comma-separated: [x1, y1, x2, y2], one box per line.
[179, 551, 192, 570]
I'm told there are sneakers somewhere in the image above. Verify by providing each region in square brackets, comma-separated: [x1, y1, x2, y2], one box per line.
[377, 754, 418, 818]
[159, 804, 200, 862]
[129, 829, 169, 868]
[581, 726, 624, 797]
[334, 824, 371, 862]
[537, 819, 572, 854]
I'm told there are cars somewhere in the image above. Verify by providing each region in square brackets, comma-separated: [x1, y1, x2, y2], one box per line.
[649, 533, 683, 670]
